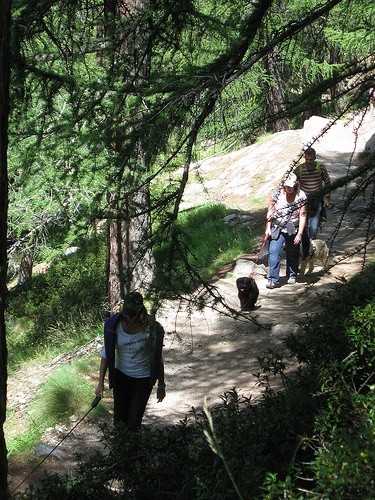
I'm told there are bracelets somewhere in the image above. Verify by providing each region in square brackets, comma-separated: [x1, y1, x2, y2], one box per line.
[157, 383, 166, 388]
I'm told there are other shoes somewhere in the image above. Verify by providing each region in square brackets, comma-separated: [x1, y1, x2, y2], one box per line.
[287, 276, 296, 284]
[266, 282, 282, 289]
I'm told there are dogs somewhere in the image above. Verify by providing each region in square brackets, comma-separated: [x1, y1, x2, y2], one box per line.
[299, 239, 330, 281]
[236, 277, 262, 311]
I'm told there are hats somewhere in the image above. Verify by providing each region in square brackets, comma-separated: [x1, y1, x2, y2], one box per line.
[122, 292, 143, 317]
[284, 173, 297, 187]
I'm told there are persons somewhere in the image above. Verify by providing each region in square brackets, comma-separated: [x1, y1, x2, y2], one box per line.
[293, 148, 332, 260]
[265, 173, 307, 289]
[95, 293, 166, 491]
